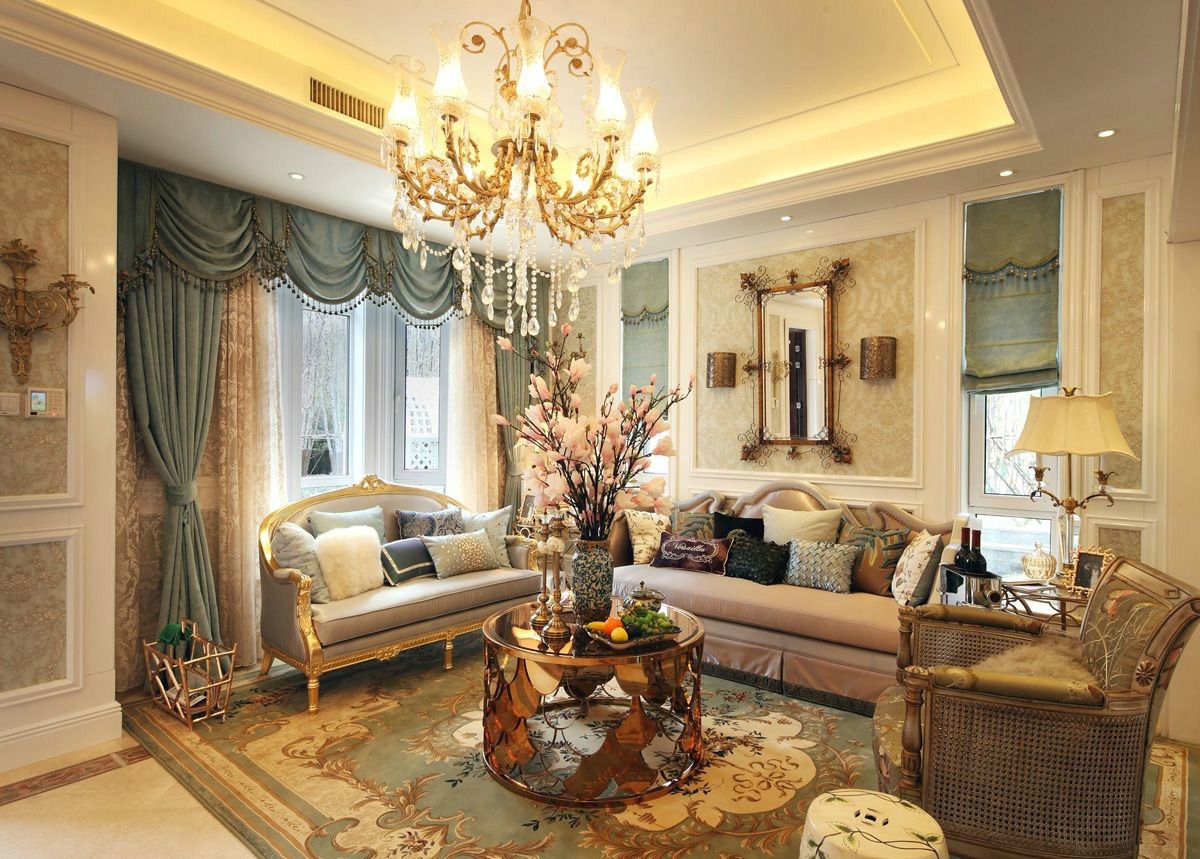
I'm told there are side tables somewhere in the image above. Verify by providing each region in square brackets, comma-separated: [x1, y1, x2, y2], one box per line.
[1003, 581, 1094, 631]
[512, 518, 580, 592]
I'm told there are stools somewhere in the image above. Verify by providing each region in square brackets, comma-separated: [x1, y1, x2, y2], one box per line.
[799, 787, 950, 859]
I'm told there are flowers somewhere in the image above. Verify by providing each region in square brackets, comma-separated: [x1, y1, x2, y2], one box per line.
[489, 322, 699, 540]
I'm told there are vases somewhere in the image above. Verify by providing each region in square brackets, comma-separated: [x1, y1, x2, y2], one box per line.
[569, 537, 616, 622]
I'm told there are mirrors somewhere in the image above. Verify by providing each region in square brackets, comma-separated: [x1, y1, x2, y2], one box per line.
[735, 255, 856, 470]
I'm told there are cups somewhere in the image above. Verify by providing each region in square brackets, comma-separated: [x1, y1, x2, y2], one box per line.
[618, 599, 633, 617]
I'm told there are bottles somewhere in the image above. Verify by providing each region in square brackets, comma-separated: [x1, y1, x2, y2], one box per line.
[1021, 543, 1057, 579]
[954, 528, 972, 569]
[964, 529, 986, 575]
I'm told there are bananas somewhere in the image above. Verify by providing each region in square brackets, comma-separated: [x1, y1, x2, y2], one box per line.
[582, 620, 603, 630]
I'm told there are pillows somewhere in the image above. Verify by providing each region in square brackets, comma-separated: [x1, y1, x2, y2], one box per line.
[762, 503, 843, 546]
[461, 503, 514, 569]
[782, 538, 859, 594]
[311, 505, 388, 547]
[722, 537, 792, 586]
[271, 521, 332, 604]
[836, 518, 913, 598]
[396, 505, 464, 540]
[381, 533, 438, 587]
[313, 525, 385, 601]
[649, 529, 734, 577]
[422, 527, 502, 579]
[713, 510, 765, 544]
[891, 528, 946, 607]
[623, 508, 671, 565]
[670, 507, 736, 543]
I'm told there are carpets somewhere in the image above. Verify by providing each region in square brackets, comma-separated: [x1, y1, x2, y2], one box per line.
[118, 624, 1199, 859]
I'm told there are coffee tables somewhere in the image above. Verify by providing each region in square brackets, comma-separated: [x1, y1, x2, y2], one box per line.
[481, 594, 705, 809]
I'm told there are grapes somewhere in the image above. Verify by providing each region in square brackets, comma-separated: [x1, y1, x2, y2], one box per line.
[622, 608, 671, 634]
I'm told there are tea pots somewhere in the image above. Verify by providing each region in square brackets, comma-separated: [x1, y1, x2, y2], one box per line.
[622, 580, 663, 614]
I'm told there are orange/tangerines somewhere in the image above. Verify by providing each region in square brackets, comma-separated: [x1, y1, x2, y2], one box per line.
[605, 617, 623, 636]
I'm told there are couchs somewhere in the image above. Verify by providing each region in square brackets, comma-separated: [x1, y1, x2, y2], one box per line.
[607, 475, 984, 720]
[871, 556, 1200, 858]
[258, 472, 551, 717]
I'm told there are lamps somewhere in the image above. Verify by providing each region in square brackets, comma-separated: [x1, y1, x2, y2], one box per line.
[0, 237, 97, 386]
[381, 1, 664, 337]
[1005, 383, 1140, 592]
[540, 498, 572, 638]
[528, 501, 555, 626]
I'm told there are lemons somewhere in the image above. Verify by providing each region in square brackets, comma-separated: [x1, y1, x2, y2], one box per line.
[611, 627, 628, 641]
